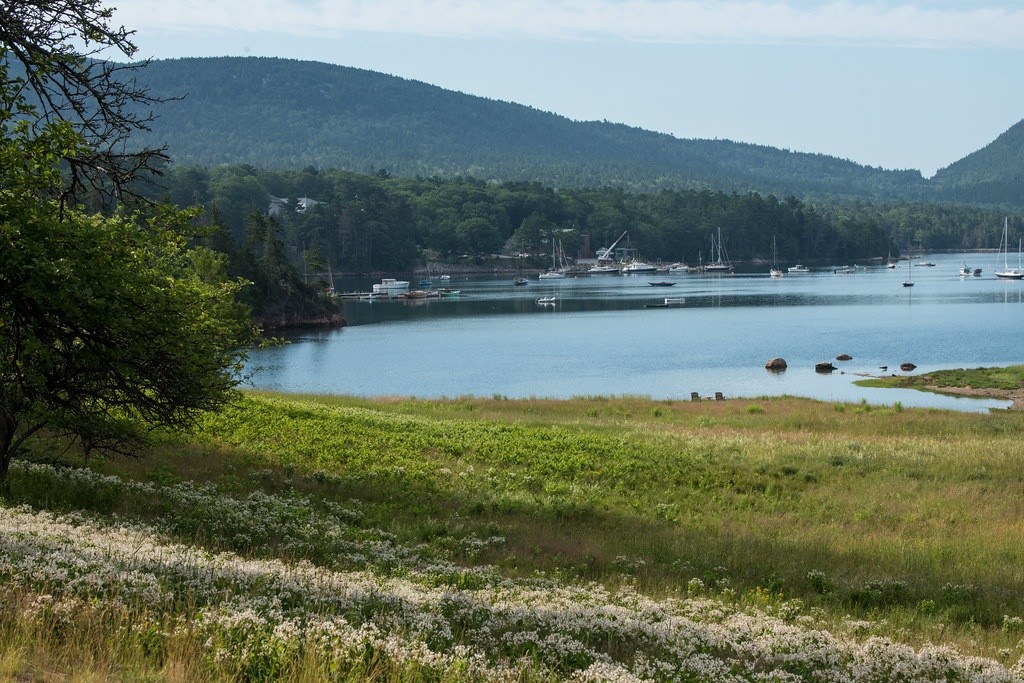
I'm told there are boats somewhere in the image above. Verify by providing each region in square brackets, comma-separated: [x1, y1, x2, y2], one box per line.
[538, 273, 565, 279]
[623, 263, 657, 273]
[439, 275, 451, 279]
[513, 279, 528, 286]
[648, 281, 677, 286]
[888, 264, 896, 269]
[440, 291, 460, 297]
[404, 291, 428, 298]
[667, 264, 688, 272]
[960, 265, 971, 275]
[419, 279, 432, 285]
[903, 281, 914, 286]
[373, 279, 409, 289]
[788, 265, 810, 273]
[535, 297, 555, 304]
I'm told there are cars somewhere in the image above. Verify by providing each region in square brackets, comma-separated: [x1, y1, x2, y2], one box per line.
[519, 253, 532, 258]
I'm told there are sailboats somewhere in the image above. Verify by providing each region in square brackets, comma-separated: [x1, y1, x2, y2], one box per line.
[770, 233, 783, 277]
[704, 227, 730, 271]
[995, 217, 1024, 280]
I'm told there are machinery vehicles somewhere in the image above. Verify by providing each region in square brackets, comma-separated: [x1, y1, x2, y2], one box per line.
[598, 231, 627, 263]
